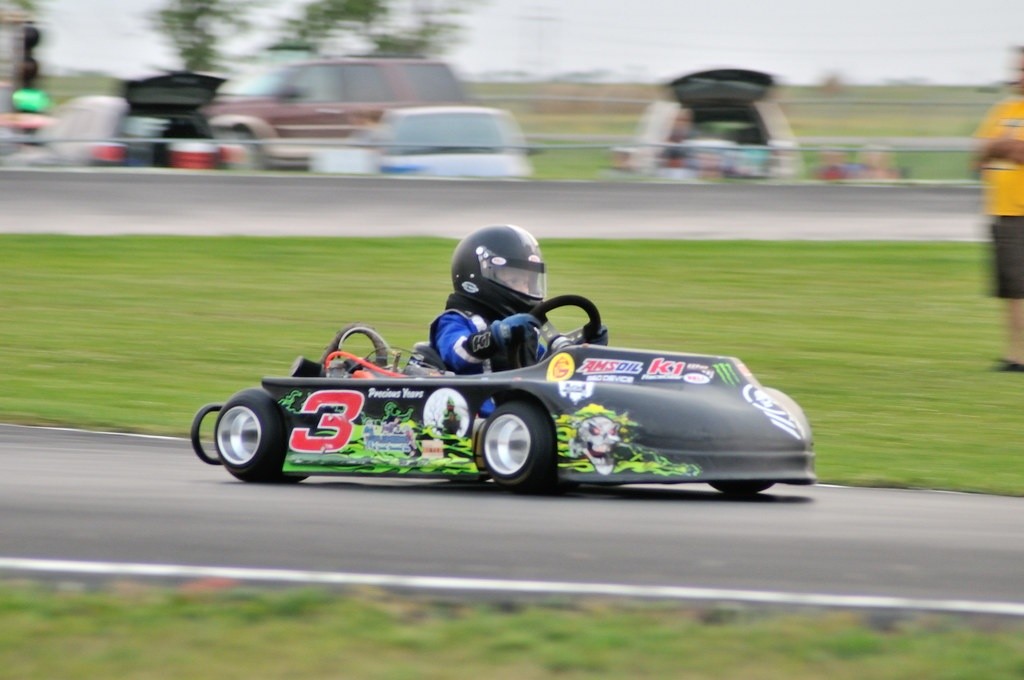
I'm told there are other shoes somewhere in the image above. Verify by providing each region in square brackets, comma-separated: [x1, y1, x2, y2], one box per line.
[990, 358, 1022, 372]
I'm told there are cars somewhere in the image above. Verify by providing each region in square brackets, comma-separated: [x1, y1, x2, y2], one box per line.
[3, 65, 249, 172]
[367, 103, 533, 182]
[624, 71, 809, 182]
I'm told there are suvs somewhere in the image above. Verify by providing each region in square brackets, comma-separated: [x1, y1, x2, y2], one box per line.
[193, 55, 453, 172]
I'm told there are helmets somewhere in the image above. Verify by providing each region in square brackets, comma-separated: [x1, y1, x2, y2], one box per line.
[453, 225, 545, 314]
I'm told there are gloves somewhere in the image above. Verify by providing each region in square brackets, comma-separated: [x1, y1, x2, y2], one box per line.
[489, 312, 546, 358]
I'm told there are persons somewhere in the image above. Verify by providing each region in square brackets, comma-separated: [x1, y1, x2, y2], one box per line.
[972, 46, 1024, 374]
[427, 224, 609, 375]
